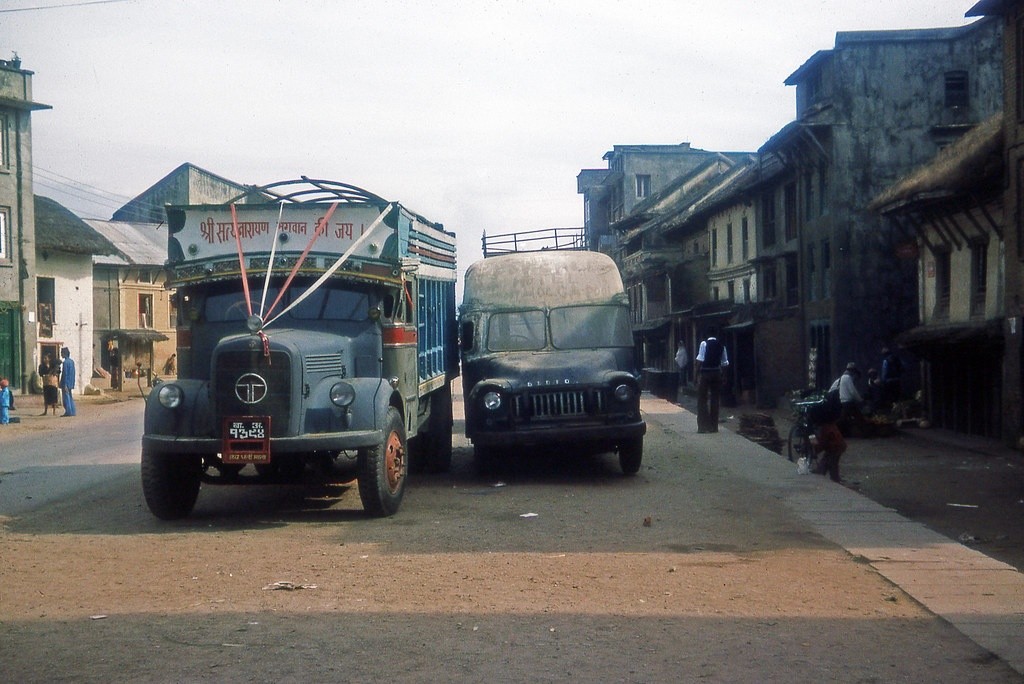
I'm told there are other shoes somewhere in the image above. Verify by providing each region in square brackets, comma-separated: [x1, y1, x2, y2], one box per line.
[698, 431, 706, 433]
[709, 430, 718, 433]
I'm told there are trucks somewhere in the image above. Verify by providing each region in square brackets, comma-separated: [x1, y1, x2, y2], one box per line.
[137, 172, 459, 520]
[454, 220, 648, 474]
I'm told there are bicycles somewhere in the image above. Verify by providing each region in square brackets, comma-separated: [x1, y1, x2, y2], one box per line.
[785, 391, 827, 469]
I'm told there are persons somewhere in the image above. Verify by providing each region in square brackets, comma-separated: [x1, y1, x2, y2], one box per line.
[942, 380, 1004, 438]
[109, 348, 118, 389]
[813, 343, 912, 481]
[696, 326, 729, 433]
[675, 340, 689, 383]
[168, 354, 176, 375]
[0, 380, 14, 424]
[60, 348, 76, 417]
[39, 351, 62, 416]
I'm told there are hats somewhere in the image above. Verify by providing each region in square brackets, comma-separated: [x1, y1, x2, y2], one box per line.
[0, 380, 8, 386]
[847, 362, 861, 374]
